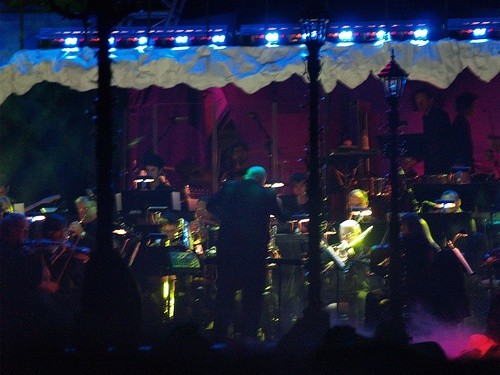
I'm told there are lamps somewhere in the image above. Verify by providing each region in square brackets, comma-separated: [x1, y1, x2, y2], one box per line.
[109, 37, 118, 54]
[171, 34, 190, 51]
[408, 28, 430, 46]
[372, 28, 394, 46]
[264, 32, 281, 48]
[210, 35, 226, 51]
[134, 36, 154, 51]
[471, 28, 489, 44]
[59, 36, 79, 53]
[336, 30, 354, 48]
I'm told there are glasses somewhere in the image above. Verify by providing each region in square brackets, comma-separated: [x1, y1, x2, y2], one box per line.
[18, 225, 28, 232]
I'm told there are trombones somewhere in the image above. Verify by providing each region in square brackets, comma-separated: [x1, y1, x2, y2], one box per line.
[154, 217, 184, 317]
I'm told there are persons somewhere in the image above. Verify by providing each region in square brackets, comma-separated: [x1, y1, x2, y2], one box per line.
[0, 180, 45, 286]
[130, 158, 175, 207]
[476, 149, 500, 180]
[449, 91, 478, 170]
[0, 309, 303, 374]
[411, 88, 452, 175]
[324, 186, 500, 374]
[284, 173, 330, 221]
[203, 163, 284, 328]
[325, 138, 358, 213]
[57, 197, 115, 272]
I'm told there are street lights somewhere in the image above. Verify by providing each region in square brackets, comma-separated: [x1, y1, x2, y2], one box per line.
[295, 2, 333, 375]
[378, 48, 411, 375]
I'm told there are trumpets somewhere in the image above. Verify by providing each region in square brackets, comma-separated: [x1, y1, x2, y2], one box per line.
[46, 212, 88, 268]
